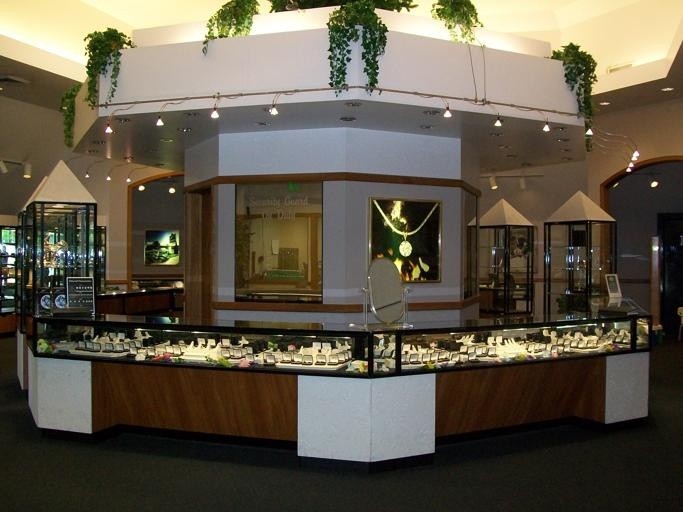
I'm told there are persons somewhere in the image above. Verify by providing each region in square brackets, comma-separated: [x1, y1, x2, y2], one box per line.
[508, 235, 527, 259]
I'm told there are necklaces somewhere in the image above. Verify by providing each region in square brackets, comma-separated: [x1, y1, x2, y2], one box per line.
[372, 199, 439, 258]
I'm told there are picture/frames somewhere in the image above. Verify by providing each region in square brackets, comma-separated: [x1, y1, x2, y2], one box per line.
[143, 228, 183, 267]
[365, 195, 444, 284]
